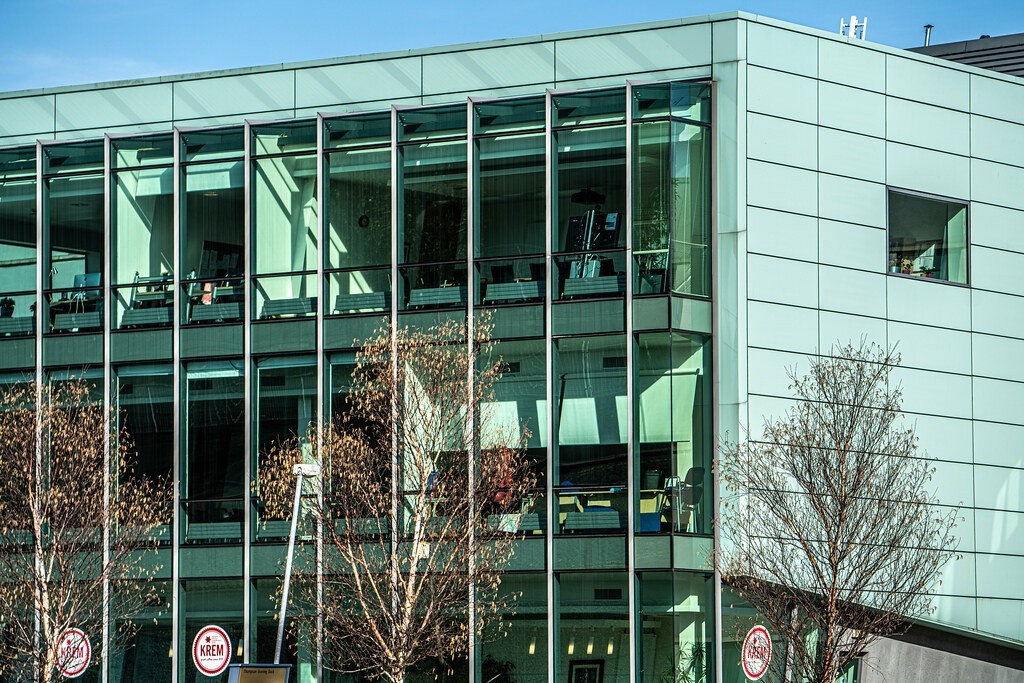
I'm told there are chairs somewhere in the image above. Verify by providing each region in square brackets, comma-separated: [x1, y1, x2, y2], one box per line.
[599, 259, 618, 276]
[556, 261, 571, 301]
[490, 265, 515, 283]
[660, 467, 706, 533]
[529, 262, 546, 281]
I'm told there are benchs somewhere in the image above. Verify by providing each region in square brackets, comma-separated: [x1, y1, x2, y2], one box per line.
[189, 302, 245, 324]
[560, 275, 626, 300]
[482, 280, 546, 305]
[0, 511, 628, 555]
[406, 286, 467, 310]
[120, 307, 174, 328]
[51, 311, 102, 335]
[332, 290, 392, 315]
[260, 297, 317, 319]
[0, 316, 36, 336]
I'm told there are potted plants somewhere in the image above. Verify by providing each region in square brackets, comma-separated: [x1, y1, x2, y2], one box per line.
[902, 262, 914, 275]
[0, 298, 15, 317]
[889, 256, 905, 273]
[919, 265, 935, 277]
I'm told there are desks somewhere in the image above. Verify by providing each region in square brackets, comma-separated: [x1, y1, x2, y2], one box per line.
[50, 296, 103, 312]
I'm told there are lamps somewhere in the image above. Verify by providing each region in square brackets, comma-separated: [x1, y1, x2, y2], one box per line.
[528, 626, 537, 655]
[568, 624, 577, 655]
[607, 626, 615, 655]
[168, 641, 172, 658]
[586, 625, 595, 654]
[237, 630, 243, 656]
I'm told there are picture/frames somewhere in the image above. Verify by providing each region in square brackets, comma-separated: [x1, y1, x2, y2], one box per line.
[568, 658, 606, 683]
